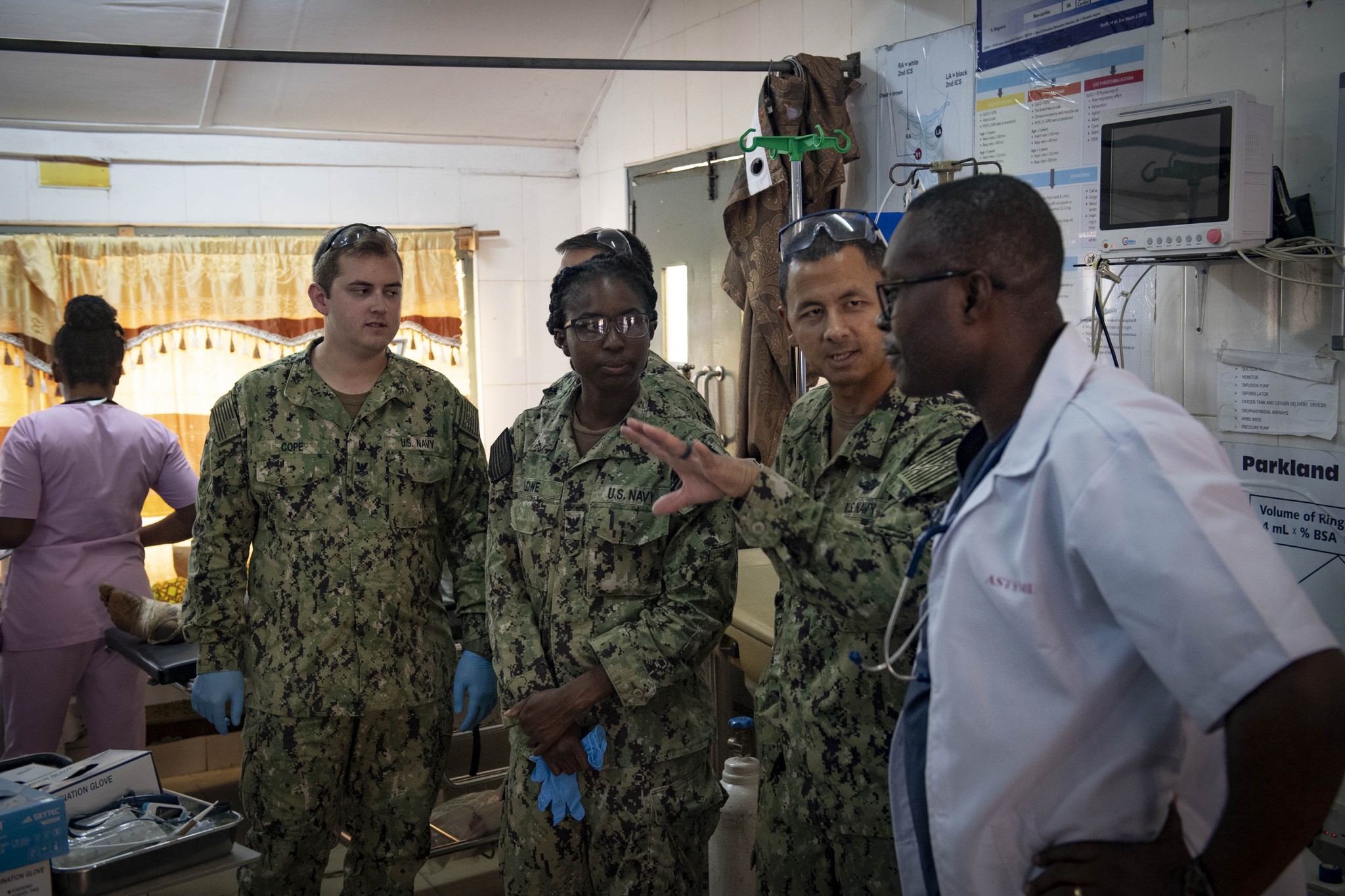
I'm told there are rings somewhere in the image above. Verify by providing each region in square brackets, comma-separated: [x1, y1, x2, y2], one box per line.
[1074, 887, 1081, 896]
[676, 443, 693, 460]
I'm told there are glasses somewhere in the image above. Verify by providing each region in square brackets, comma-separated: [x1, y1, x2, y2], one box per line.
[875, 267, 1007, 320]
[582, 227, 633, 255]
[563, 313, 650, 342]
[778, 208, 889, 264]
[321, 223, 399, 253]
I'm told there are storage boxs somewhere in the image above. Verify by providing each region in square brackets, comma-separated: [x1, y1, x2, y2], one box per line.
[0, 763, 60, 785]
[21, 749, 166, 822]
[0, 857, 53, 896]
[0, 776, 68, 875]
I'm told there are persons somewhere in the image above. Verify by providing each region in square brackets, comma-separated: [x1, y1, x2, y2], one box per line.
[538, 225, 713, 426]
[870, 173, 1344, 895]
[99, 561, 455, 645]
[186, 225, 498, 896]
[482, 255, 741, 896]
[618, 210, 980, 896]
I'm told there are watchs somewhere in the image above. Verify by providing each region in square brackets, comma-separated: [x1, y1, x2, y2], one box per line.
[0, 294, 196, 773]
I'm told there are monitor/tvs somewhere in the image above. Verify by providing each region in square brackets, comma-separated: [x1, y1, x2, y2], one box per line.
[1096, 91, 1274, 253]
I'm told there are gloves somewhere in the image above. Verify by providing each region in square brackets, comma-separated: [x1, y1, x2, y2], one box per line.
[529, 724, 607, 826]
[191, 670, 243, 734]
[453, 650, 498, 732]
